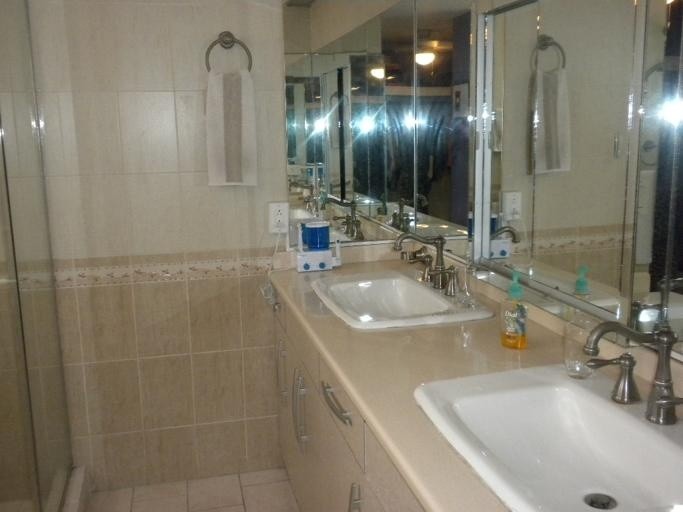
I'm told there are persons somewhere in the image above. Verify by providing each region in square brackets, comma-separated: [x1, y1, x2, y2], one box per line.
[356, 101, 469, 223]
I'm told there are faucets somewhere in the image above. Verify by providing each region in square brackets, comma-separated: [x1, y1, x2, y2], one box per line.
[659, 275, 683, 325]
[398, 194, 428, 216]
[329, 180, 351, 194]
[321, 195, 358, 214]
[392, 232, 448, 289]
[581, 317, 677, 423]
[491, 224, 523, 246]
[289, 182, 315, 195]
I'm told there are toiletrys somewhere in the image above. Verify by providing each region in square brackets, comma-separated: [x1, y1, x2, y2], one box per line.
[573, 266, 594, 299]
[499, 270, 527, 349]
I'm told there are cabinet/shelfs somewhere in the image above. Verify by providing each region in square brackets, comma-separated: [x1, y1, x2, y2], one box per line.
[261, 288, 420, 512]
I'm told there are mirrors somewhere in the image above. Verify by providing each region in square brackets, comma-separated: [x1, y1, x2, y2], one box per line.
[483, 0, 683, 362]
[284, 0, 478, 269]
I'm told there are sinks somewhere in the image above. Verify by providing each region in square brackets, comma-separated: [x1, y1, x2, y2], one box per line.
[480, 258, 615, 312]
[288, 206, 318, 221]
[331, 228, 347, 243]
[416, 362, 683, 512]
[310, 268, 494, 330]
[599, 299, 683, 348]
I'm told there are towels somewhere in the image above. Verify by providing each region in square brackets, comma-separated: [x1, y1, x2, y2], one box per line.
[205, 71, 257, 188]
